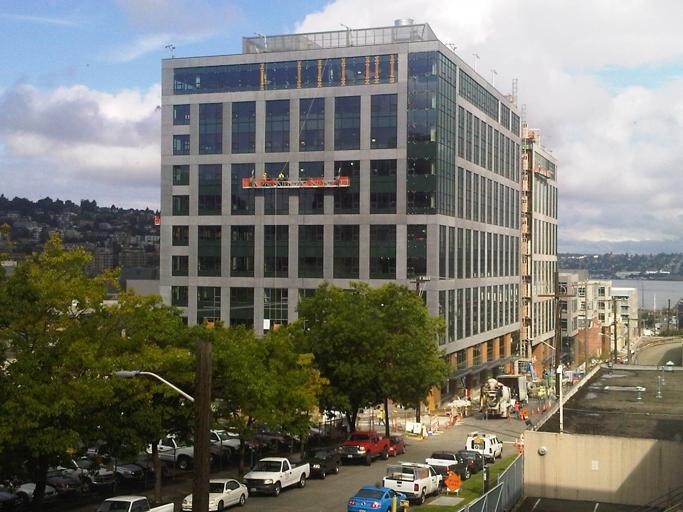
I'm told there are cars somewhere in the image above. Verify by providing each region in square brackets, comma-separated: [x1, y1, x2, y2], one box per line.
[181, 478, 249, 512]
[384, 435, 406, 456]
[211, 422, 331, 464]
[456, 450, 487, 474]
[298, 447, 341, 479]
[0, 416, 195, 511]
[346, 482, 408, 512]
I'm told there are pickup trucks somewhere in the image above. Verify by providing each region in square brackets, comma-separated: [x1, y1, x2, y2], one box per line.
[338, 430, 390, 466]
[382, 461, 444, 506]
[426, 451, 467, 486]
[241, 456, 311, 497]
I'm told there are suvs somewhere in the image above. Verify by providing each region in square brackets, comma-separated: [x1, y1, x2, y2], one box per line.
[464, 431, 503, 462]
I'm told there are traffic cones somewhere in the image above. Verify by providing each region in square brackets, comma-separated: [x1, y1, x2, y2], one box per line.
[530, 404, 545, 417]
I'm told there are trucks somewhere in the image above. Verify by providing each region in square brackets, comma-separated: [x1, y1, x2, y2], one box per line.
[496, 374, 528, 407]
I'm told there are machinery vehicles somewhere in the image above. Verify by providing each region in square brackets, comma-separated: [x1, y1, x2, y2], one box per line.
[478, 378, 514, 418]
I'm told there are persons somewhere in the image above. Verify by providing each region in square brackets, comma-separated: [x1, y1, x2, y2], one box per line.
[377, 409, 386, 426]
[262, 172, 267, 186]
[513, 401, 520, 420]
[279, 173, 284, 185]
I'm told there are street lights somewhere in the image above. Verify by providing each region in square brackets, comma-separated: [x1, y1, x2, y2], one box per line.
[528, 337, 561, 397]
[115, 342, 212, 512]
[597, 333, 617, 364]
